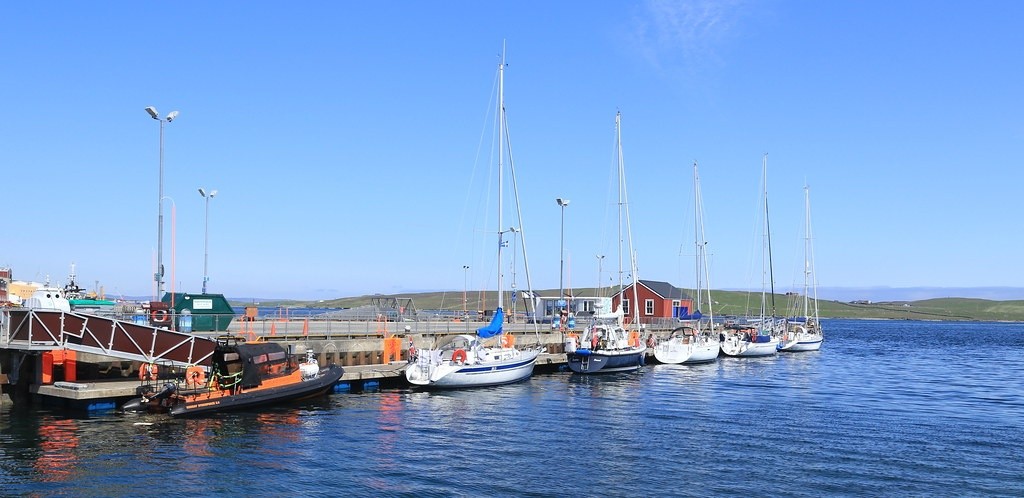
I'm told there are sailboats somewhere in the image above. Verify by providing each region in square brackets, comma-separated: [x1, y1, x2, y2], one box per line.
[721, 154, 779, 358]
[567, 109, 647, 373]
[403, 38, 543, 389]
[776, 182, 824, 351]
[653, 158, 721, 364]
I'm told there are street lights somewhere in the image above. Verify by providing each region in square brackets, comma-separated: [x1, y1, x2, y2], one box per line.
[694, 241, 708, 317]
[146, 106, 179, 301]
[462, 265, 469, 315]
[197, 188, 217, 293]
[595, 254, 605, 318]
[557, 198, 571, 319]
[509, 227, 520, 324]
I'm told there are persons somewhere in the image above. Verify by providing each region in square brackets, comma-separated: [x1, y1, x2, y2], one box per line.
[560, 308, 568, 330]
[593, 329, 603, 351]
[647, 336, 654, 348]
[568, 311, 576, 318]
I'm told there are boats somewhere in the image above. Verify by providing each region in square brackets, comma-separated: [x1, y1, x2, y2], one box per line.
[120, 341, 346, 415]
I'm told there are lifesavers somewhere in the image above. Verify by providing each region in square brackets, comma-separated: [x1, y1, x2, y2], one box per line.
[152, 309, 168, 324]
[139, 363, 158, 381]
[186, 366, 206, 386]
[450, 350, 468, 364]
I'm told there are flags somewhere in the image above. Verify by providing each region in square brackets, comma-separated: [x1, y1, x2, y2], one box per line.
[409, 338, 415, 355]
